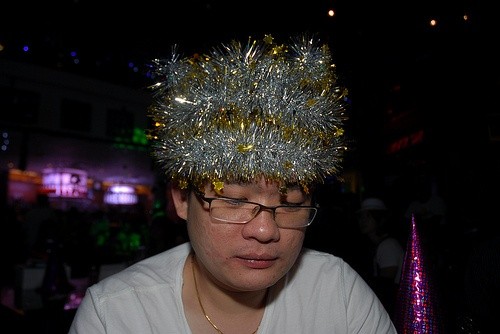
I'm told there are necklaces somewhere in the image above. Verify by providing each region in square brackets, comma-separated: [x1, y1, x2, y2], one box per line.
[190, 252, 267, 334]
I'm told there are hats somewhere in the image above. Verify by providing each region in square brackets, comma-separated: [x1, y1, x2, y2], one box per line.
[357, 199, 386, 212]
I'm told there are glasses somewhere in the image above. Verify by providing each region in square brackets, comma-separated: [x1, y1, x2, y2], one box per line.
[188, 180, 318, 229]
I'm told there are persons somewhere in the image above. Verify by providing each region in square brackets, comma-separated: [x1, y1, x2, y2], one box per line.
[68, 39, 400, 334]
